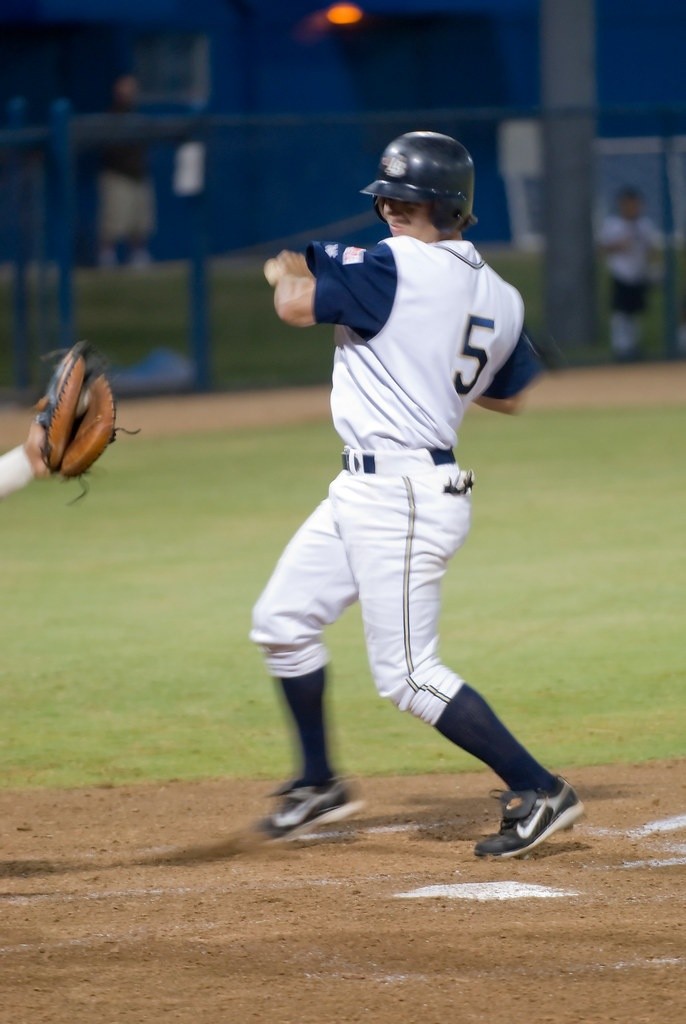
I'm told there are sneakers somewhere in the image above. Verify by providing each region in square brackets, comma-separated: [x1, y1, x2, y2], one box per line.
[474, 780, 584, 861]
[261, 774, 366, 844]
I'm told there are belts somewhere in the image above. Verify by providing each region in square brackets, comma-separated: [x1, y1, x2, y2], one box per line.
[339, 448, 461, 473]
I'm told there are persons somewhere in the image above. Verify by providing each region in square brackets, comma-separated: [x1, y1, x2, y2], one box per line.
[94, 75, 193, 270]
[0, 402, 76, 499]
[247, 130, 587, 860]
[595, 186, 667, 363]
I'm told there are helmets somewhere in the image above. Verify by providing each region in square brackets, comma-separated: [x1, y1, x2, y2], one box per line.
[360, 130, 477, 232]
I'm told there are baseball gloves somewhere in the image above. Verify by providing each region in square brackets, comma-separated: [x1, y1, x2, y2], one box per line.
[23, 338, 142, 509]
